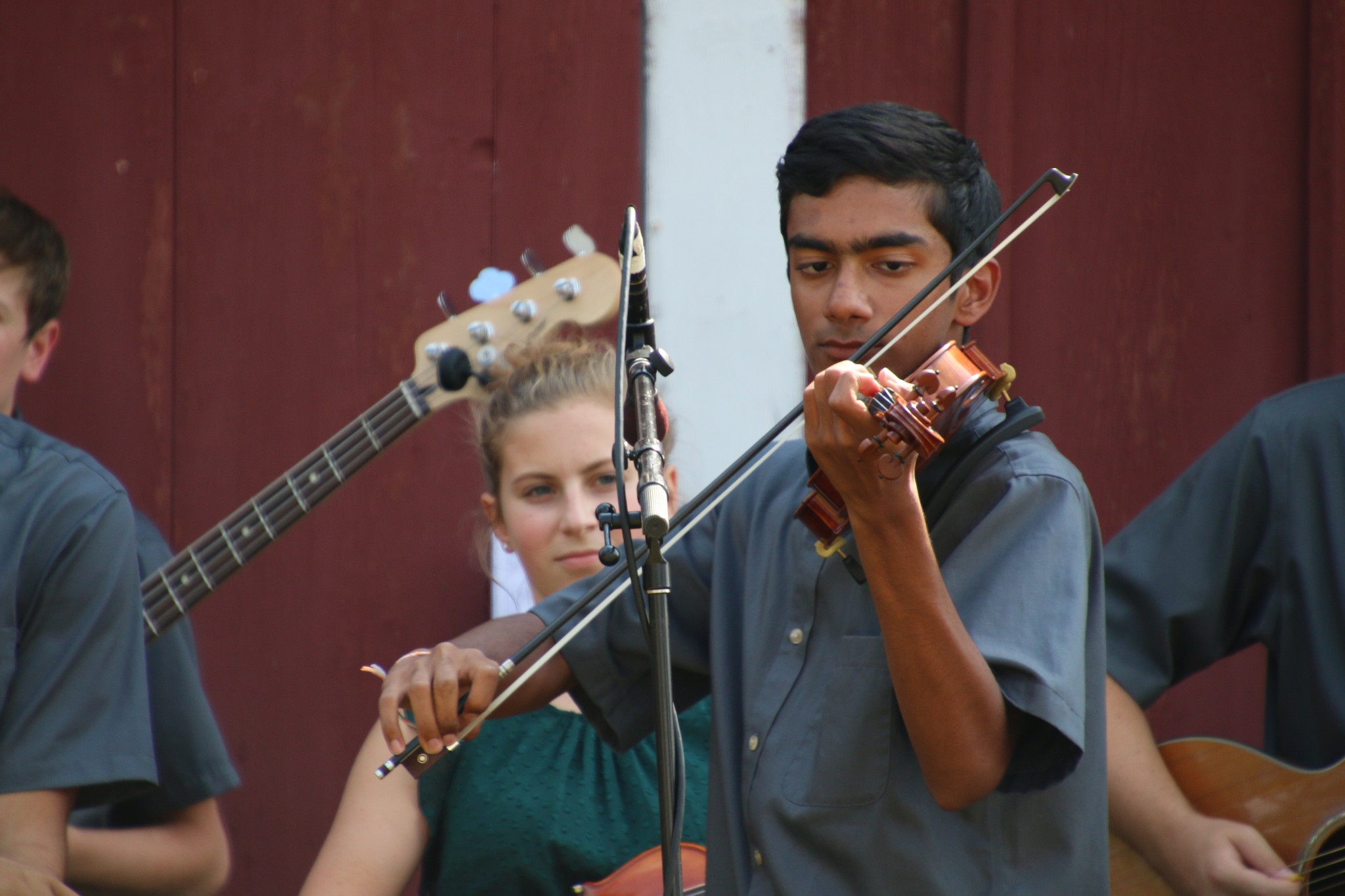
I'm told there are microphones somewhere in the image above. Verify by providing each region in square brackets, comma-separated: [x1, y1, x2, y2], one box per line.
[617, 218, 668, 446]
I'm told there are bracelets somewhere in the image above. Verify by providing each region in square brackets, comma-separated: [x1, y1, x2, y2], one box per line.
[360, 646, 431, 730]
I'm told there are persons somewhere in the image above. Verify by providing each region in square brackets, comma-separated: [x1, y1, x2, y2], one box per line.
[299, 336, 714, 896]
[0, 191, 241, 896]
[1104, 374, 1345, 896]
[378, 100, 1110, 896]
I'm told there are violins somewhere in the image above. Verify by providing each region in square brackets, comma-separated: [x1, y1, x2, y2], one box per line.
[790, 338, 1008, 551]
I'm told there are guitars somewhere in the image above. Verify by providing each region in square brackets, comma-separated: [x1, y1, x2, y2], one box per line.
[1107, 735, 1345, 896]
[581, 841, 707, 896]
[137, 222, 627, 648]
